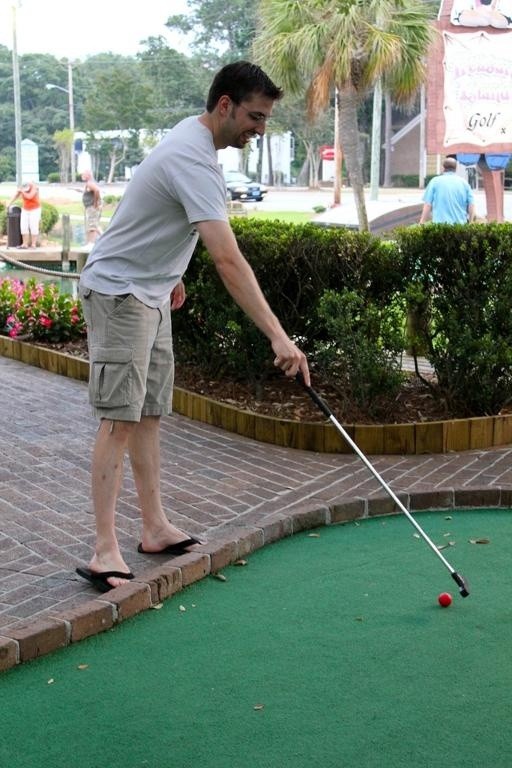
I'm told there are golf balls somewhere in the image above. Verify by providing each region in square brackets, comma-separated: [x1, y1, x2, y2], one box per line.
[439, 593, 452, 606]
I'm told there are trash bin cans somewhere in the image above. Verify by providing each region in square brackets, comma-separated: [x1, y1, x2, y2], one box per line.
[8, 206, 22, 247]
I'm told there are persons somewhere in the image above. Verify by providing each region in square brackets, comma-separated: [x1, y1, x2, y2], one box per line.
[81, 168, 104, 246]
[6, 179, 43, 249]
[418, 157, 476, 227]
[77, 60, 312, 592]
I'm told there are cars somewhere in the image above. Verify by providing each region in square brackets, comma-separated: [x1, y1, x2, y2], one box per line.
[224, 172, 267, 201]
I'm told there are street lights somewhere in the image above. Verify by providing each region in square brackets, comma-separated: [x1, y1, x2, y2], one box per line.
[46, 83, 76, 183]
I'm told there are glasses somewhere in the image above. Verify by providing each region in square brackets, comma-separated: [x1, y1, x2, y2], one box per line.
[240, 104, 268, 124]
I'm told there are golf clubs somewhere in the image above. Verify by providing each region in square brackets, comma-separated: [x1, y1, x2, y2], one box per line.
[296, 367, 470, 597]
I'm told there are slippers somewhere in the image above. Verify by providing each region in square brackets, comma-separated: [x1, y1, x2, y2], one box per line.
[136, 536, 202, 555]
[75, 565, 135, 593]
